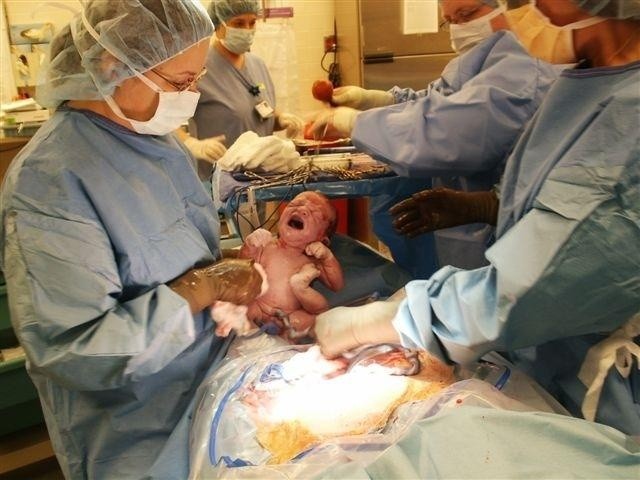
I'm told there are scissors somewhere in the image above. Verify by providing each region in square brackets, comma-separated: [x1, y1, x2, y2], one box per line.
[243, 165, 363, 190]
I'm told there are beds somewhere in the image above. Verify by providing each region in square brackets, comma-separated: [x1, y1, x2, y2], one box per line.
[252, 232, 639, 421]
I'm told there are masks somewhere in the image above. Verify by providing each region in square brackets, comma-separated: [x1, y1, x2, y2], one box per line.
[217, 23, 256, 55]
[449, 9, 500, 56]
[500, 0, 575, 64]
[95, 74, 202, 136]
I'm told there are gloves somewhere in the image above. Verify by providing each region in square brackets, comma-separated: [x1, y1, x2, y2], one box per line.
[184, 134, 227, 164]
[279, 110, 304, 131]
[303, 85, 394, 139]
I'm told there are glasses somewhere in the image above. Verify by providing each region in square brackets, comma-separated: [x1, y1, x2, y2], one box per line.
[151, 67, 208, 95]
[440, 4, 491, 32]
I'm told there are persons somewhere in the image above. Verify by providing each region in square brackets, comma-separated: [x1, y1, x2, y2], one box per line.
[307, 0, 578, 272]
[315, 0, 640, 436]
[174, 0, 304, 180]
[238, 191, 343, 345]
[0, 0, 263, 480]
